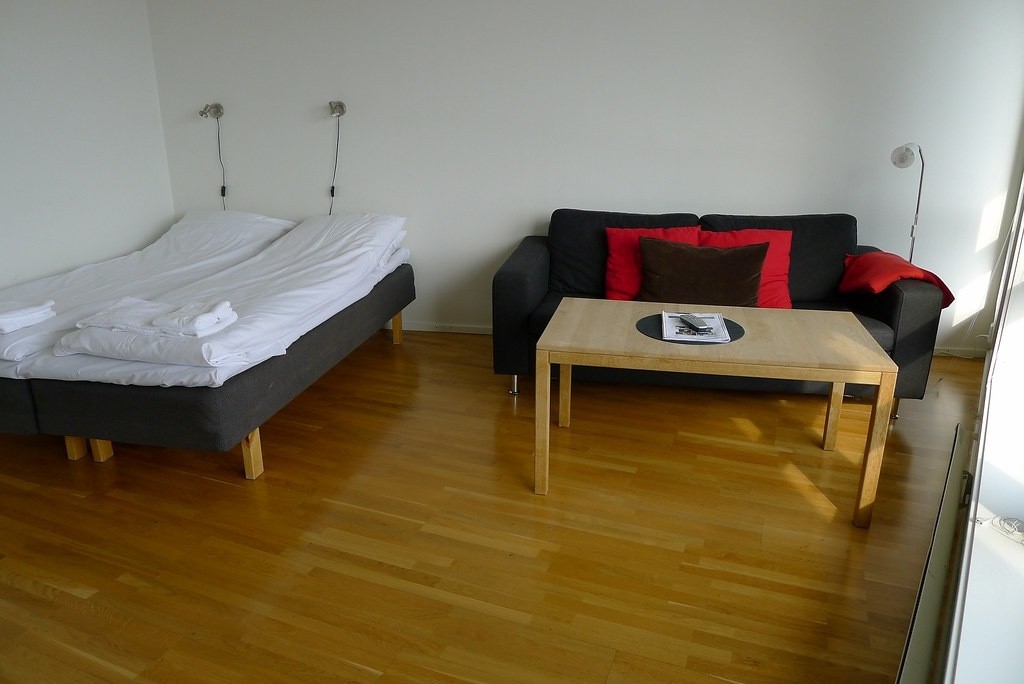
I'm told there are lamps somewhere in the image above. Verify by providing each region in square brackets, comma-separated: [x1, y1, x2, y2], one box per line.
[889, 143, 925, 264]
[198, 102, 224, 120]
[329, 101, 347, 117]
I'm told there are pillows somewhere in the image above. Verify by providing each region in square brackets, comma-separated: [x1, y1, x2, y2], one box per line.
[840, 253, 955, 309]
[603, 224, 701, 303]
[631, 236, 771, 308]
[695, 228, 793, 309]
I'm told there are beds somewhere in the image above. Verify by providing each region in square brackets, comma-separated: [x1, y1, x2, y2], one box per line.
[0, 213, 416, 480]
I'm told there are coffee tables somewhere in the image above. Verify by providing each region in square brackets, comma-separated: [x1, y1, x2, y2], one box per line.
[535, 297, 899, 528]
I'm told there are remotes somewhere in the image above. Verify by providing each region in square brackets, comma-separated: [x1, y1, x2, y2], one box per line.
[680, 314, 708, 332]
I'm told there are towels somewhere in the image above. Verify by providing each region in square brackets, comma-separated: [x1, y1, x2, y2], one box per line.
[74, 295, 237, 338]
[0, 299, 57, 335]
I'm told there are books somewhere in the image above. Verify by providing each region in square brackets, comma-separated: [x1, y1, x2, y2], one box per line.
[662, 310, 731, 343]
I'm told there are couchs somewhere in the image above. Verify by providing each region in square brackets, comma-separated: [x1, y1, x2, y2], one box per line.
[492, 208, 943, 420]
